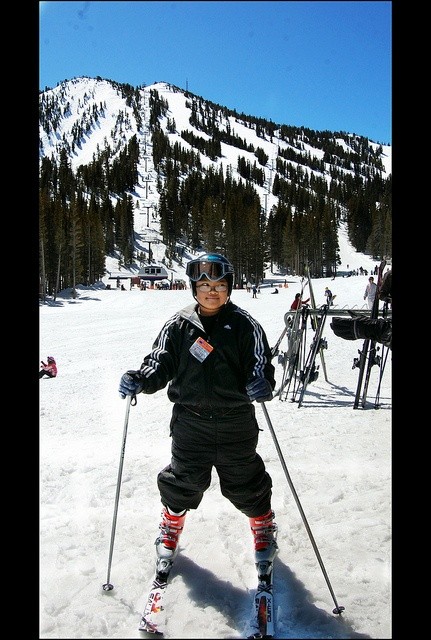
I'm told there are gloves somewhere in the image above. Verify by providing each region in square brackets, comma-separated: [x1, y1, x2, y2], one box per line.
[119, 369, 146, 400]
[245, 375, 274, 404]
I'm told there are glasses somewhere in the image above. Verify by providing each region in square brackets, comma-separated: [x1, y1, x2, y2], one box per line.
[195, 285, 228, 294]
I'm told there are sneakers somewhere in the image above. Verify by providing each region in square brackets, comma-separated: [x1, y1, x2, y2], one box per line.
[155, 506, 186, 564]
[248, 514, 277, 562]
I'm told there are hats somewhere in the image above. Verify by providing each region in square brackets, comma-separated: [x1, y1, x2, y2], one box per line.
[47, 355, 54, 361]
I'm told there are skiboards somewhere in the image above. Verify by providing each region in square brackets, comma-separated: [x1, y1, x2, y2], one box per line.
[139, 522, 279, 640]
[297, 295, 336, 408]
[268, 280, 308, 399]
[352, 259, 387, 409]
[303, 267, 328, 383]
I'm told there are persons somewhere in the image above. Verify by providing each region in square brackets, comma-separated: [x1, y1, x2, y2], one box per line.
[363, 277, 377, 309]
[290, 293, 310, 311]
[39, 356, 57, 379]
[119, 253, 278, 563]
[323, 287, 333, 306]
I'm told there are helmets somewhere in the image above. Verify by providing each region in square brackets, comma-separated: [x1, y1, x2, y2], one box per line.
[186, 255, 235, 298]
